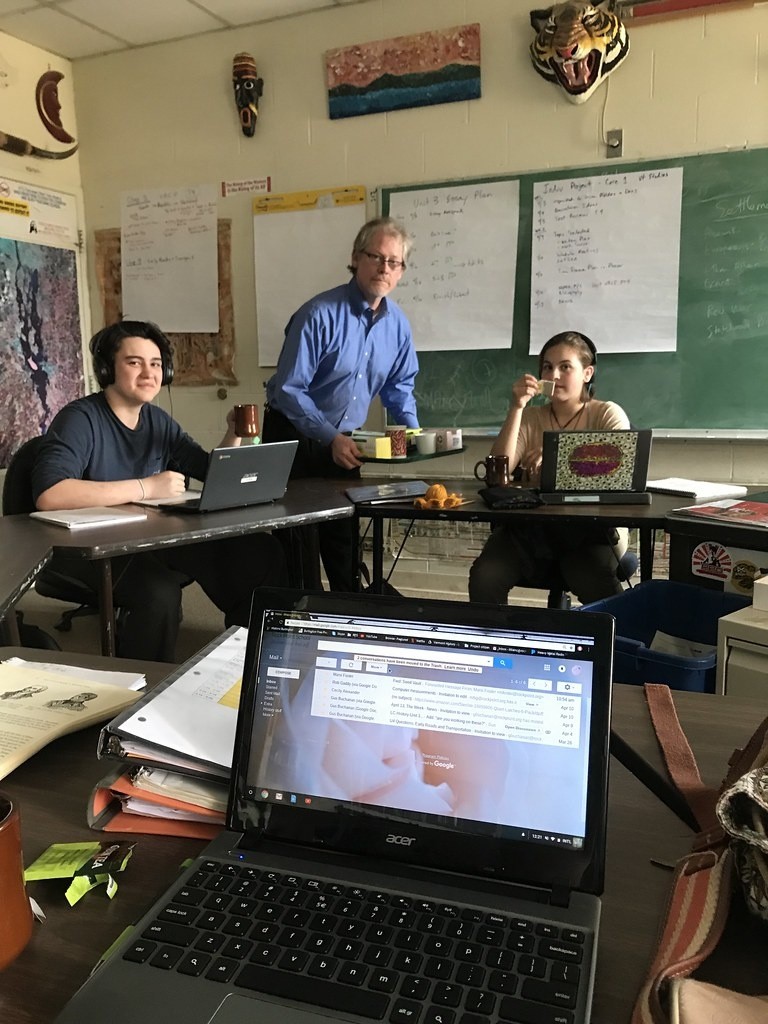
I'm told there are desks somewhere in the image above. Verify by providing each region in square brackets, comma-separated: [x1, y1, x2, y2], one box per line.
[0, 475, 768, 1024]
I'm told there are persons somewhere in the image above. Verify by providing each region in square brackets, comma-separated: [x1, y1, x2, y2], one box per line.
[469, 331, 630, 606]
[31, 316, 292, 663]
[262, 214, 419, 594]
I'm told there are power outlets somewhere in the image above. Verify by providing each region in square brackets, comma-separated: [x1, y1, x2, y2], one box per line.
[606, 127, 624, 159]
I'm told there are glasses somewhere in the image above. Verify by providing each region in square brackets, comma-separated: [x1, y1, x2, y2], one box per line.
[359, 250, 403, 271]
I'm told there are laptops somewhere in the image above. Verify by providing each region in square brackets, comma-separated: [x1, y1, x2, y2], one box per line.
[52, 588, 616, 1024]
[158, 440, 300, 514]
[528, 430, 652, 494]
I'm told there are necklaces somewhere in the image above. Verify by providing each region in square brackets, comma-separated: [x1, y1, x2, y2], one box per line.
[550, 405, 584, 429]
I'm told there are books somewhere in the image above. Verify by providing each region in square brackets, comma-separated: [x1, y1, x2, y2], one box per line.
[87, 624, 249, 839]
[31, 507, 149, 528]
[0, 656, 147, 780]
[645, 478, 747, 499]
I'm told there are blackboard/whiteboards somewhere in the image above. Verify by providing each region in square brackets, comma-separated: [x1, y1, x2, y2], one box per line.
[371, 144, 768, 444]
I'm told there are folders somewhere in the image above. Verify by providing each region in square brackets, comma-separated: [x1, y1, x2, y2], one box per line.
[96, 624, 248, 786]
[86, 762, 226, 840]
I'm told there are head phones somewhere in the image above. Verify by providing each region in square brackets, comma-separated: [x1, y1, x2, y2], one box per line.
[93, 320, 175, 388]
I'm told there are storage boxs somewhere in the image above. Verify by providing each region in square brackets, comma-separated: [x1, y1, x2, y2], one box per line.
[573, 577, 751, 693]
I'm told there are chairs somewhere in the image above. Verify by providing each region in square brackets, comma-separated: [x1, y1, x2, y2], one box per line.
[491, 424, 638, 610]
[1, 433, 183, 633]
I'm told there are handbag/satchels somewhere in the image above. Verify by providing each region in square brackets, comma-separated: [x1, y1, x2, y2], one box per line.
[635, 681, 768, 1024]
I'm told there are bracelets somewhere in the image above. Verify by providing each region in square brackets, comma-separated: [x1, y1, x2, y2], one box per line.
[138, 478, 145, 501]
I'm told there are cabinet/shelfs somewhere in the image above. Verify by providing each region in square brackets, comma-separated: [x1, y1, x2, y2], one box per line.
[715, 603, 768, 700]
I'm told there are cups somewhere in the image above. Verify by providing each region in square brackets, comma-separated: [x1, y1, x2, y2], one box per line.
[415, 432, 436, 454]
[385, 425, 406, 459]
[234, 404, 259, 437]
[0, 790, 32, 974]
[474, 455, 509, 487]
